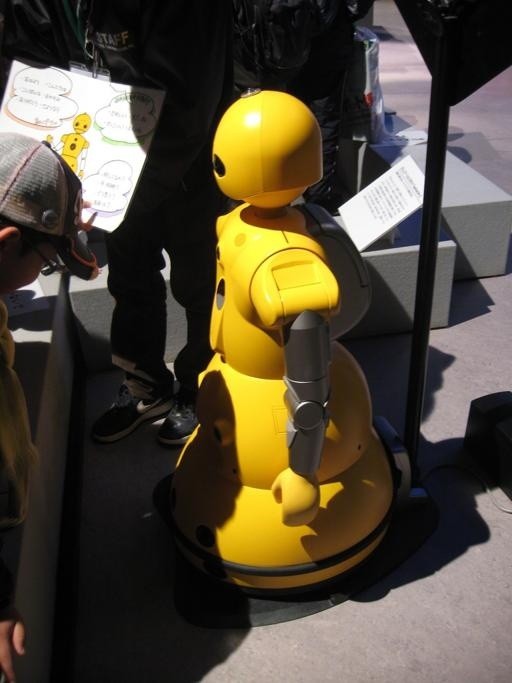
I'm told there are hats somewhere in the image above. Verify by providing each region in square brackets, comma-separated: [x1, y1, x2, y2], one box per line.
[0, 131, 99, 280]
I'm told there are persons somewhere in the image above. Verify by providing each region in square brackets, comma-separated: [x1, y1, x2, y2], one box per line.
[230, 0, 379, 215]
[1, 130, 103, 682]
[0, 0, 240, 449]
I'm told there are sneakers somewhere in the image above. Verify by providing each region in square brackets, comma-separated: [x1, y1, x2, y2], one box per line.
[158, 392, 200, 446]
[91, 384, 175, 443]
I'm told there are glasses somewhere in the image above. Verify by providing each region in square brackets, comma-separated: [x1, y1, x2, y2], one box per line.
[32, 245, 59, 276]
